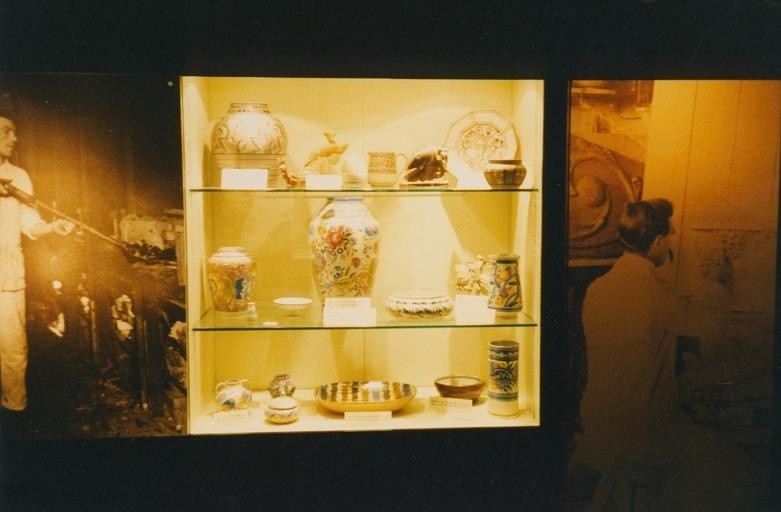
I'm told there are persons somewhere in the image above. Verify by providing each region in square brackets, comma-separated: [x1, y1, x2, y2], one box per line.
[578, 200, 762, 512]
[0, 115, 74, 412]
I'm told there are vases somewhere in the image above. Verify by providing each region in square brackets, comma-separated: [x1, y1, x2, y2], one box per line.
[307, 195, 382, 310]
[205, 246, 254, 317]
[211, 101, 288, 187]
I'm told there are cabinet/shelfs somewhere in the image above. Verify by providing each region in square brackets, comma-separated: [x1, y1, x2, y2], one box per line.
[177, 72, 544, 436]
